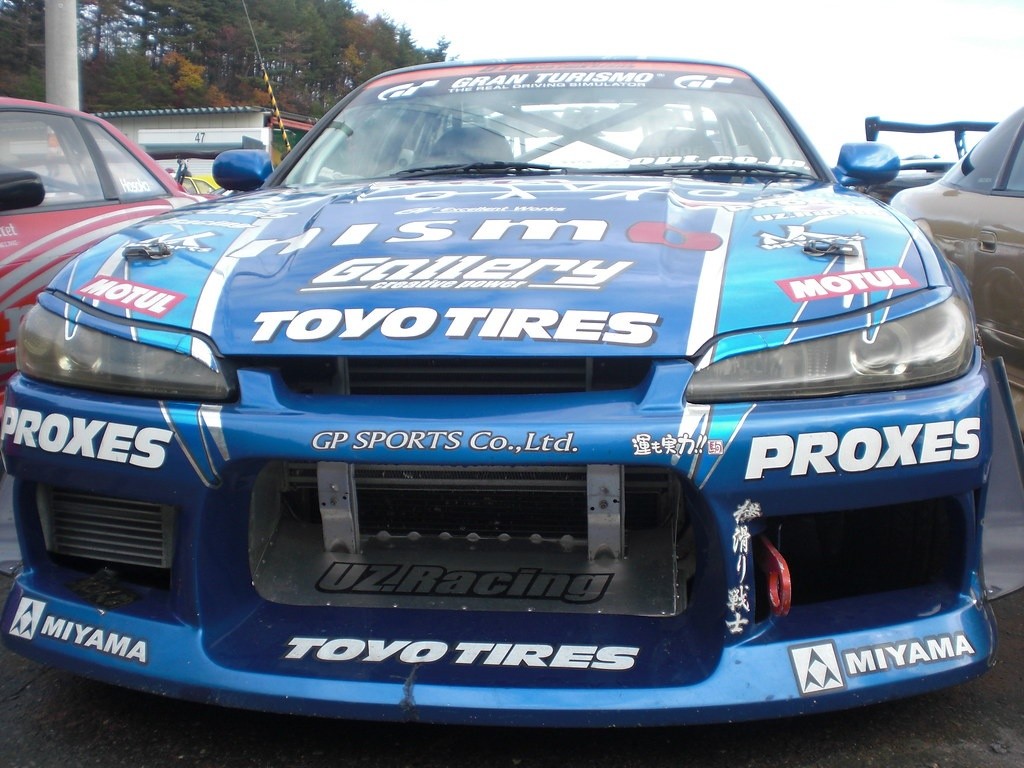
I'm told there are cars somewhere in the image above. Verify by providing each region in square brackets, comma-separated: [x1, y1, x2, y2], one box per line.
[845, 103, 1024, 448]
[0, 54, 1001, 737]
[0, 93, 230, 465]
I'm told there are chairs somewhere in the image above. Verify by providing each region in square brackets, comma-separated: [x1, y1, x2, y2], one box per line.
[430, 124, 515, 164]
[632, 129, 719, 158]
